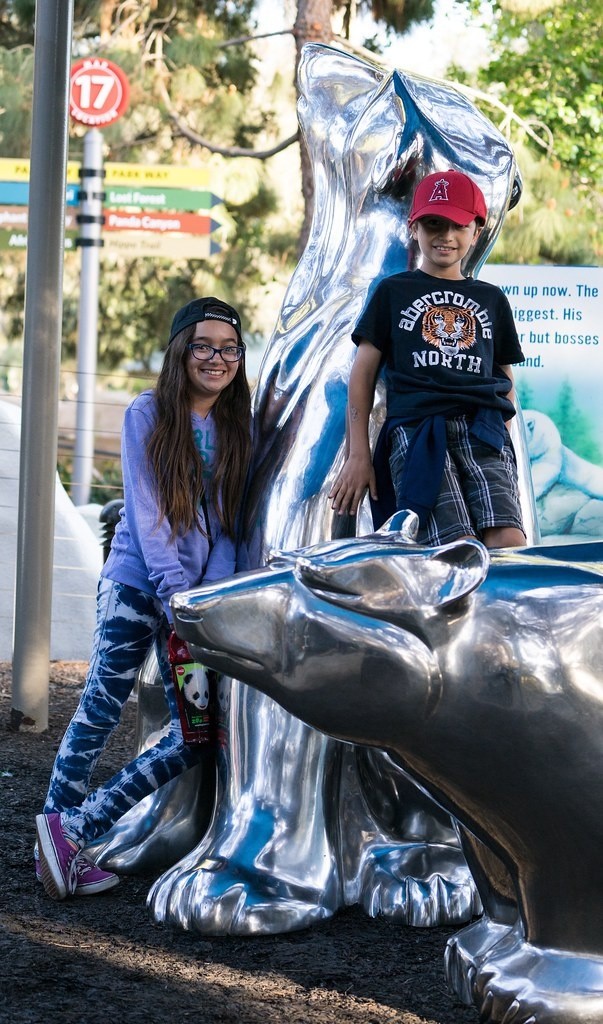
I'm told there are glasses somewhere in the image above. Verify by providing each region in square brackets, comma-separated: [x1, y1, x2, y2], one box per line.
[184, 339, 244, 364]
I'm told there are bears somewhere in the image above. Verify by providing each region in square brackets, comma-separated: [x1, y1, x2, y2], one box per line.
[168, 506, 603, 954]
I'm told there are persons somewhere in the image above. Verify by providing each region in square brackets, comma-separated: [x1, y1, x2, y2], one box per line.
[326, 169, 527, 548]
[33, 297, 257, 901]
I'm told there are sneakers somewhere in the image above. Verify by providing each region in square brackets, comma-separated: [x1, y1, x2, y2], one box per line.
[32, 813, 120, 898]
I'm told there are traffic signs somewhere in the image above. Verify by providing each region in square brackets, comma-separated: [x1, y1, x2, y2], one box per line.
[0, 156, 223, 262]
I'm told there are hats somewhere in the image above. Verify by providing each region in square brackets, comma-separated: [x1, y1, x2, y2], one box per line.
[407, 169, 487, 230]
[168, 296, 241, 339]
